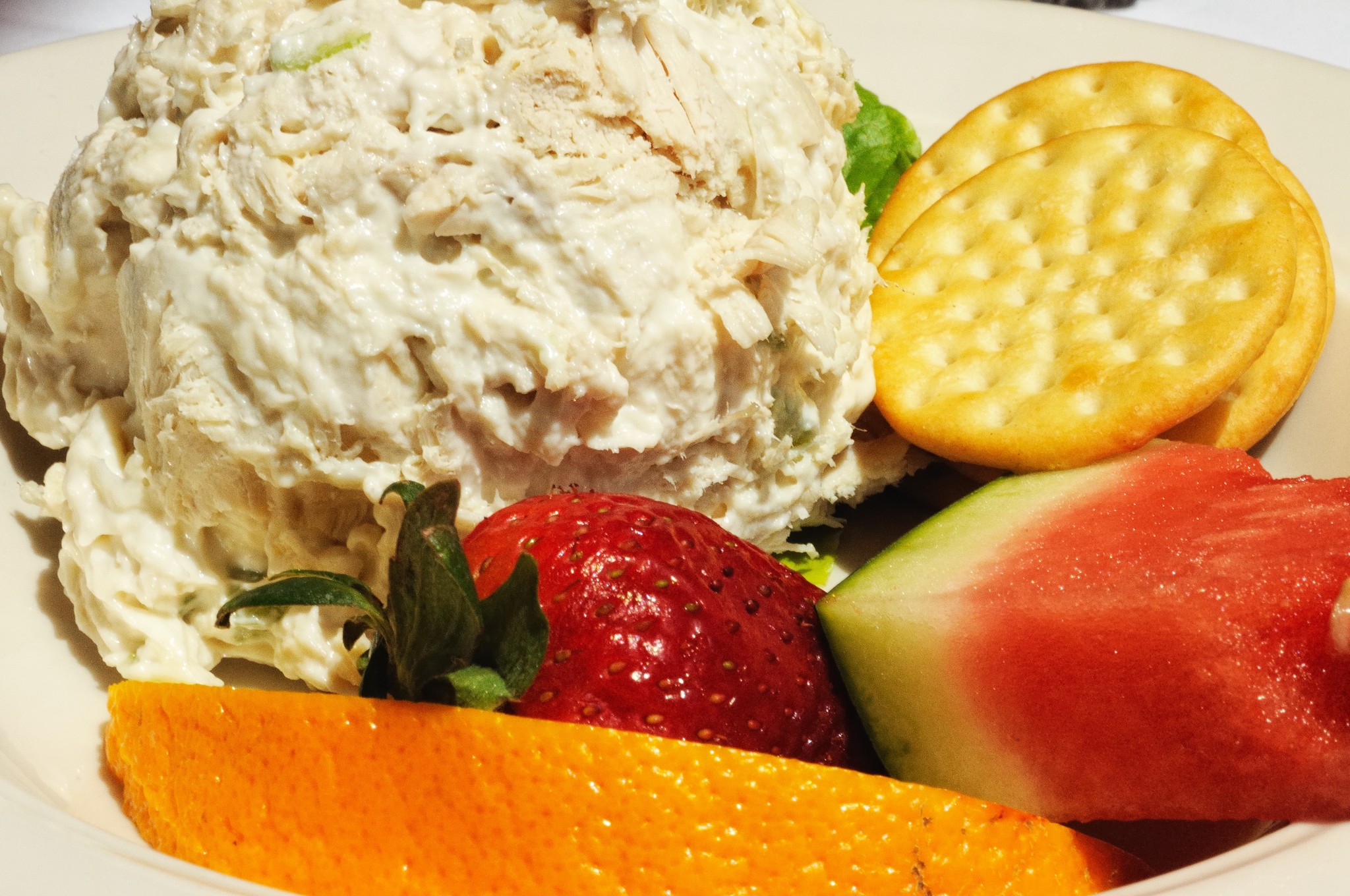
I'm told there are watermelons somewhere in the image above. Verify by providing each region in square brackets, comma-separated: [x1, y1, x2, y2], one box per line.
[816, 440, 1350, 823]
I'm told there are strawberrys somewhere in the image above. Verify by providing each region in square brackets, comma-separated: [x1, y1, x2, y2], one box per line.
[217, 478, 852, 769]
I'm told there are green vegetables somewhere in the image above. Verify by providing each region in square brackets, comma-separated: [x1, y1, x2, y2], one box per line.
[841, 83, 922, 242]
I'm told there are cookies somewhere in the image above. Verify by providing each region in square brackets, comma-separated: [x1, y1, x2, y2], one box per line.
[870, 62, 1336, 473]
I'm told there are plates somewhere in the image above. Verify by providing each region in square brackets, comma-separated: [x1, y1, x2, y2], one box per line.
[0, 0, 1350, 896]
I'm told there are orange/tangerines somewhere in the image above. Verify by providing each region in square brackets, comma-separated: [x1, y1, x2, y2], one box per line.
[101, 681, 1142, 896]
